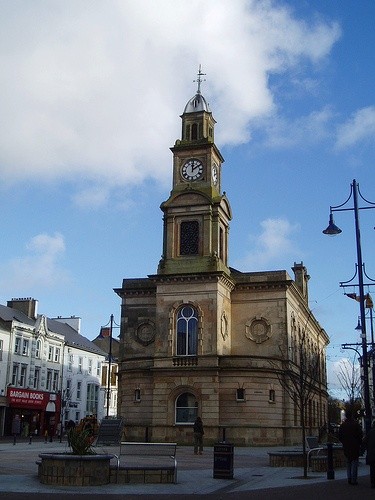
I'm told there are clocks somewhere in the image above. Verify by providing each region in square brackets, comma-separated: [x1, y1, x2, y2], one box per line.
[211, 165, 218, 185]
[181, 159, 203, 182]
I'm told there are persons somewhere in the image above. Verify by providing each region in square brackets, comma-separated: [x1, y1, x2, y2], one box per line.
[193, 416, 205, 455]
[337, 412, 375, 487]
[44, 430, 48, 444]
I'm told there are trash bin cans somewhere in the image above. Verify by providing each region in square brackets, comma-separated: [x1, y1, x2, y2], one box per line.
[213, 442, 235, 479]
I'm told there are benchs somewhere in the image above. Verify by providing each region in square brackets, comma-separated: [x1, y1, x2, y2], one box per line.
[110, 442, 179, 486]
[96, 419, 124, 445]
[305, 436, 328, 472]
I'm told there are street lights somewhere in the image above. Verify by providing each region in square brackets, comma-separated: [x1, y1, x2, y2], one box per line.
[320, 178, 375, 425]
[96, 314, 120, 419]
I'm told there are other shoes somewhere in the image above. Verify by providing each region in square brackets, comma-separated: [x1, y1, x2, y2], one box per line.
[199, 451, 203, 455]
[195, 452, 198, 455]
